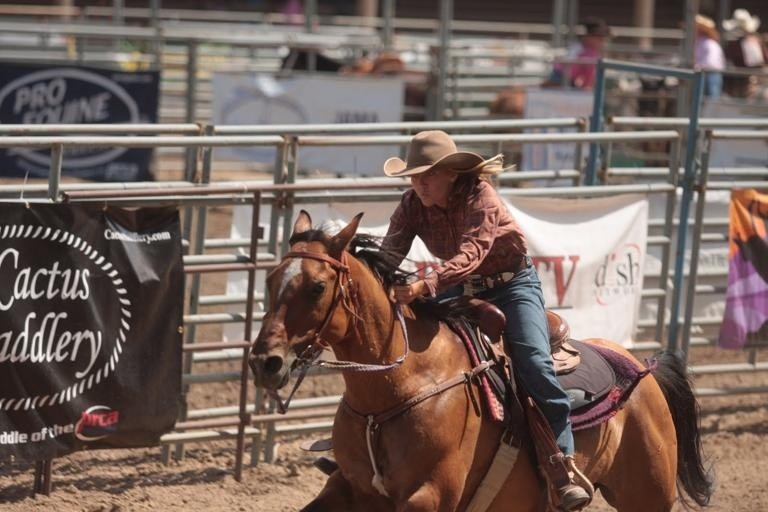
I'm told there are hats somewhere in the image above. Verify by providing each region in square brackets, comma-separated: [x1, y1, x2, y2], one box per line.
[575, 16, 613, 39]
[679, 13, 721, 42]
[383, 130, 486, 180]
[722, 9, 762, 38]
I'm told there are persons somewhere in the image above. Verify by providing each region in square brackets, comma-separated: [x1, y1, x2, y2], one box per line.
[370, 130, 592, 510]
[721, 9, 768, 100]
[539, 16, 613, 89]
[670, 14, 725, 96]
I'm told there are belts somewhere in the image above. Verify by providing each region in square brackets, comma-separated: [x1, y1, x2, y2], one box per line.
[458, 257, 535, 293]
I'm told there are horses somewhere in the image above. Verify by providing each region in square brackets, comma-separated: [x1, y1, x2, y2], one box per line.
[487, 85, 527, 152]
[282, 46, 429, 128]
[636, 73, 676, 169]
[246, 208, 715, 512]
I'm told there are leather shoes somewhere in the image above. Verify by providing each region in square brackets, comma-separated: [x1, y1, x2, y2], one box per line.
[540, 481, 590, 510]
[313, 455, 339, 476]
